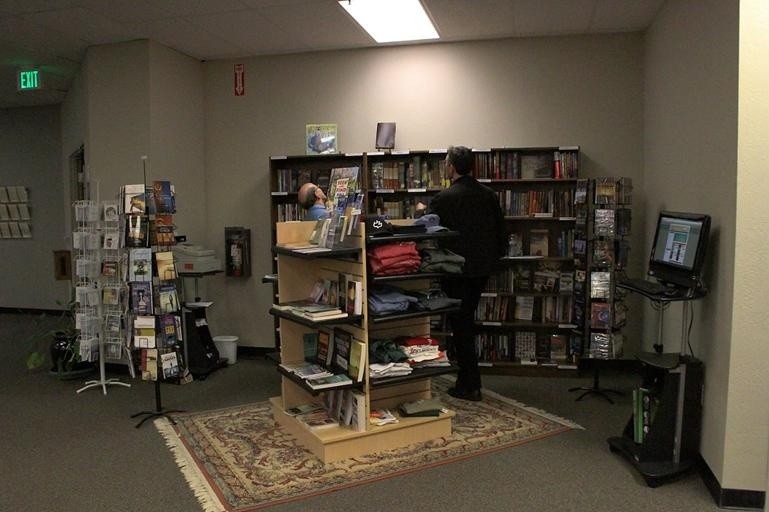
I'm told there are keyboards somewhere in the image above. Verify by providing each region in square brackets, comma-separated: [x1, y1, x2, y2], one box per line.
[622, 277, 664, 295]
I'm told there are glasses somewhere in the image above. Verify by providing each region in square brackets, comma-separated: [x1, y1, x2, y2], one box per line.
[312, 184, 320, 192]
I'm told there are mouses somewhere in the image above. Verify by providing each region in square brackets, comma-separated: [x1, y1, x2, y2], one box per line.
[665, 287, 680, 296]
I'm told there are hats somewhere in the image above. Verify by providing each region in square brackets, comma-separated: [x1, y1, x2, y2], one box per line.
[415, 213, 449, 234]
[367, 214, 394, 236]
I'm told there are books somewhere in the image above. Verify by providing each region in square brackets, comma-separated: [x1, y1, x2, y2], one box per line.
[474, 331, 571, 362]
[376, 122, 396, 148]
[310, 216, 349, 249]
[305, 123, 339, 154]
[507, 221, 574, 258]
[273, 270, 364, 322]
[274, 164, 366, 222]
[470, 152, 579, 218]
[278, 326, 366, 391]
[69, 181, 222, 383]
[483, 260, 573, 295]
[367, 158, 453, 220]
[475, 294, 573, 325]
[0, 185, 32, 239]
[282, 388, 365, 428]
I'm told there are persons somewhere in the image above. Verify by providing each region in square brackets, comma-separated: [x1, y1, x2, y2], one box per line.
[413, 144, 511, 403]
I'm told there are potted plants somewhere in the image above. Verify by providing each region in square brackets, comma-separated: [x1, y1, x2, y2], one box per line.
[23, 294, 96, 380]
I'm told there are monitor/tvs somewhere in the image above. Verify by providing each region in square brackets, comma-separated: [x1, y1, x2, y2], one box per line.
[647, 210, 711, 288]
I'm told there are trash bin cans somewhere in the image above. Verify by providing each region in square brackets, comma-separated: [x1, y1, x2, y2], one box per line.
[212, 335, 239, 364]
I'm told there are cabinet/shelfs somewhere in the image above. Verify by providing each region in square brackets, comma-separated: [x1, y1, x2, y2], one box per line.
[264, 146, 589, 377]
[561, 178, 632, 405]
[608, 274, 709, 486]
[261, 200, 458, 465]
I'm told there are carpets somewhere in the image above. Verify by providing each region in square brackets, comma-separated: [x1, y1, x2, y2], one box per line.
[149, 374, 587, 512]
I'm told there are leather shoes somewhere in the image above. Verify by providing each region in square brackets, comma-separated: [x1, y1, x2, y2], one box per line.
[447, 386, 482, 402]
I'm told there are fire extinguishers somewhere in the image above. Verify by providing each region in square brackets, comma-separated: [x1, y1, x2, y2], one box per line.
[230, 240, 242, 277]
